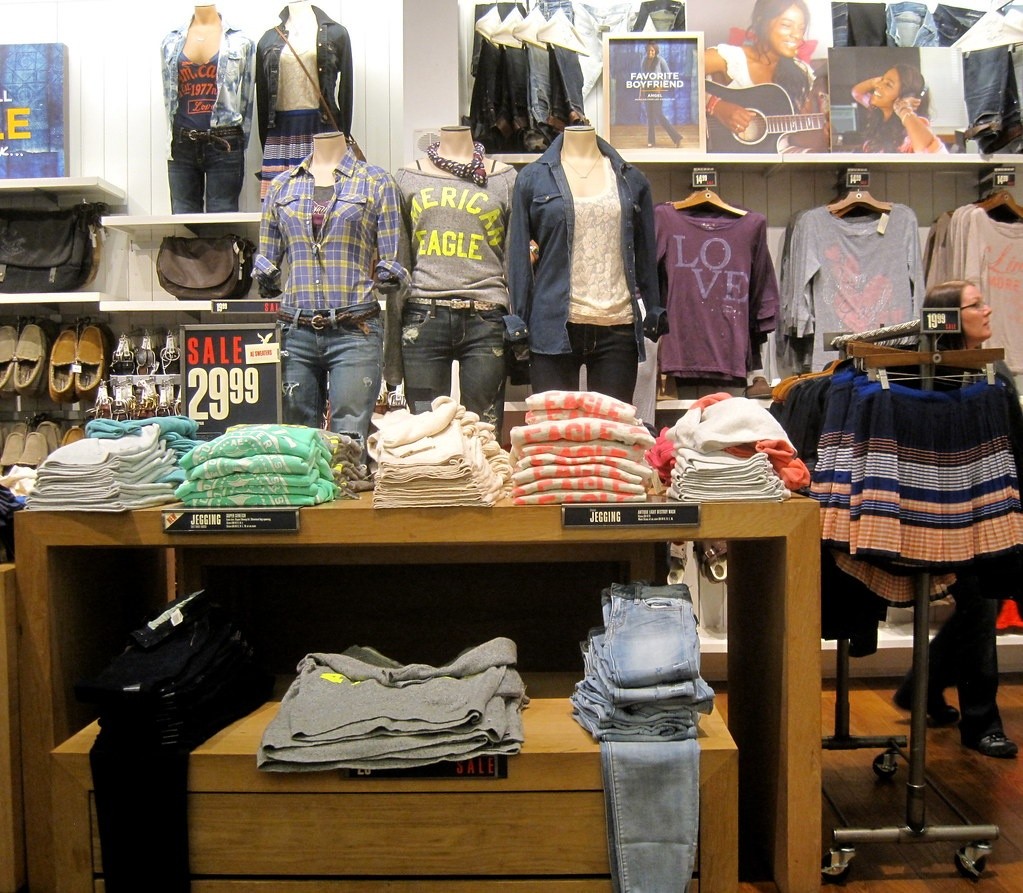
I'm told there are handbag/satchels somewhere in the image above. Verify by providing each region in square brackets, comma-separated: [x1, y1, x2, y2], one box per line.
[0, 204, 108, 293]
[156, 237, 257, 301]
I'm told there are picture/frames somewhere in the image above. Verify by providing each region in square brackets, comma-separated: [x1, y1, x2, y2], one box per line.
[827, 46, 967, 154]
[684, 0, 833, 153]
[602, 31, 708, 154]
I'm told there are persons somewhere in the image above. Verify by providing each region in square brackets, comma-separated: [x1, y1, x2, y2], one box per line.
[502, 127, 669, 407]
[160, 4, 257, 216]
[892, 282, 1021, 760]
[390, 127, 523, 447]
[703, 1, 830, 153]
[254, 0, 353, 212]
[637, 42, 683, 148]
[851, 65, 950, 156]
[249, 131, 410, 473]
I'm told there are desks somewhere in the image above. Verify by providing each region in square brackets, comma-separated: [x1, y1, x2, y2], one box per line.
[12, 487, 823, 891]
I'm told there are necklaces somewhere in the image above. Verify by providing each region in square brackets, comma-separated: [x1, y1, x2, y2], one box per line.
[561, 152, 602, 179]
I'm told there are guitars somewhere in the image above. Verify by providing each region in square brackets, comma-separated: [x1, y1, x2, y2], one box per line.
[705, 81, 828, 153]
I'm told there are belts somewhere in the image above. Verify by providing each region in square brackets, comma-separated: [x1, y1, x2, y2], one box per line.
[173, 125, 243, 152]
[407, 297, 502, 311]
[276, 307, 380, 329]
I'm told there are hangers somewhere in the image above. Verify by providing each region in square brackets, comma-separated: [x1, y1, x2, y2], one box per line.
[770, 320, 1005, 404]
[490, 0, 529, 49]
[474, 0, 507, 50]
[654, 167, 1023, 220]
[536, 0, 590, 57]
[512, 0, 556, 52]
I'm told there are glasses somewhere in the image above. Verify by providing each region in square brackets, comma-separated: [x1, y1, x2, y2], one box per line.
[961, 300, 986, 311]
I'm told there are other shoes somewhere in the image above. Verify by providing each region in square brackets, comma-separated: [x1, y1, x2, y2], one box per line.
[0, 319, 115, 403]
[1, 422, 85, 475]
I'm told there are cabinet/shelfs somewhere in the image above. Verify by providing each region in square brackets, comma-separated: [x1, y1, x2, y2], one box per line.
[0, 174, 403, 316]
[47, 664, 743, 892]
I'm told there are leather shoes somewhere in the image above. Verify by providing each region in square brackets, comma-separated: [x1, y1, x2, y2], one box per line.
[893, 690, 960, 724]
[960, 730, 1018, 757]
